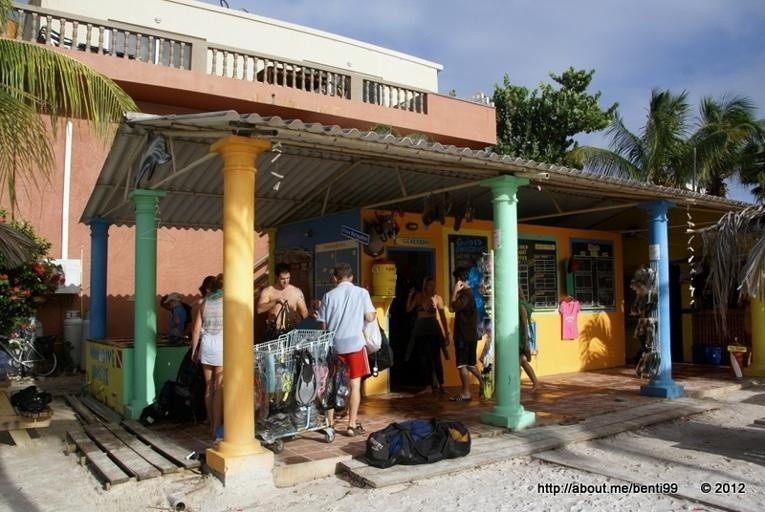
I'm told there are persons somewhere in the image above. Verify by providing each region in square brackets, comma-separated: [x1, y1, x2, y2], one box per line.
[191, 273, 224, 440]
[406, 275, 450, 395]
[519, 287, 540, 395]
[257, 263, 309, 421]
[448, 267, 486, 402]
[192, 276, 215, 425]
[160, 294, 192, 340]
[316, 262, 377, 436]
[308, 268, 350, 418]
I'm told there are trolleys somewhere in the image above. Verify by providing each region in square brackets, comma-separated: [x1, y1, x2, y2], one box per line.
[252, 327, 337, 454]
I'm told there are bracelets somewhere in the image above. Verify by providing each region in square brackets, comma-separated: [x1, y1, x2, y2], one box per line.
[445, 331, 450, 335]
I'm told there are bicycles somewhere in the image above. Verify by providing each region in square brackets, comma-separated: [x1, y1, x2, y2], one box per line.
[1, 331, 58, 376]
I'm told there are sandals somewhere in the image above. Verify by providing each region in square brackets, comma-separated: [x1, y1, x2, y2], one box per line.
[346, 422, 366, 438]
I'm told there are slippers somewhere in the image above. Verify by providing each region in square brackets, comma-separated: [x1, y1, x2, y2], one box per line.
[449, 394, 472, 401]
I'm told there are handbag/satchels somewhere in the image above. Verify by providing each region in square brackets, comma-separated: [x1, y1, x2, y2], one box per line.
[11, 386, 53, 414]
[368, 329, 395, 376]
[159, 381, 207, 426]
[366, 418, 471, 469]
[293, 352, 352, 411]
